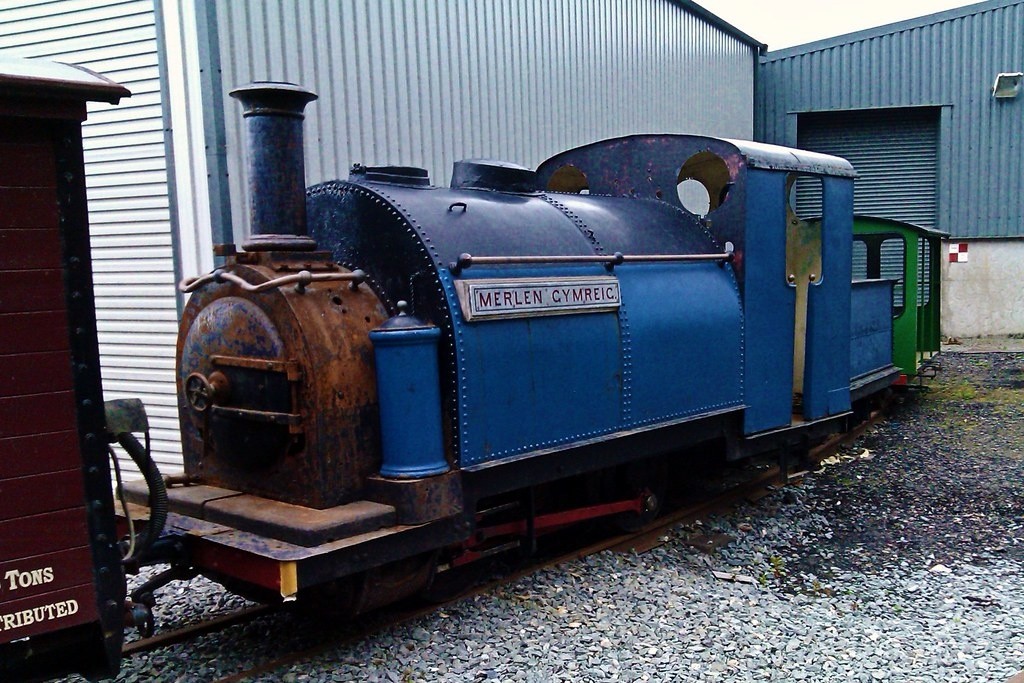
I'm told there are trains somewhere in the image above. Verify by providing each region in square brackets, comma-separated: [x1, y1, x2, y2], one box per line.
[0, 38, 953, 683]
